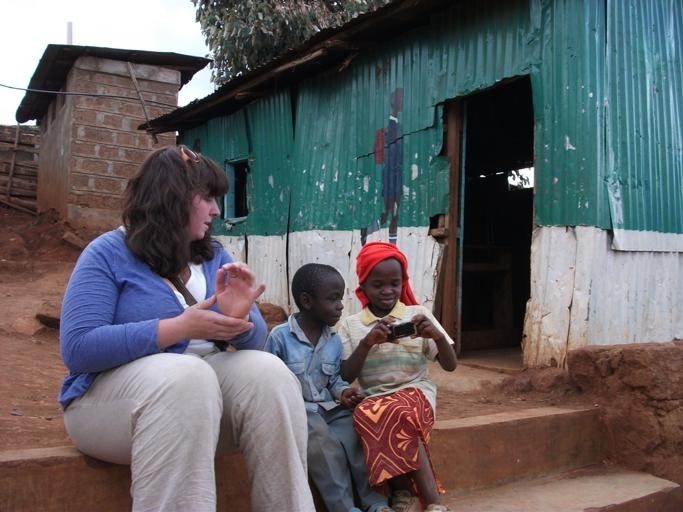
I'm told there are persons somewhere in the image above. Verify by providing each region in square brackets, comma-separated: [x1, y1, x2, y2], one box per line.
[360, 89, 405, 245]
[263, 263, 393, 511]
[59, 144, 319, 512]
[337, 243, 457, 511]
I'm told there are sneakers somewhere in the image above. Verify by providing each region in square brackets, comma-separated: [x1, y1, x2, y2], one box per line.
[391, 490, 420, 512]
[423, 503, 447, 512]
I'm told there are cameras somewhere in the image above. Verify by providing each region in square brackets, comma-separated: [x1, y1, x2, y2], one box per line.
[387, 320, 418, 339]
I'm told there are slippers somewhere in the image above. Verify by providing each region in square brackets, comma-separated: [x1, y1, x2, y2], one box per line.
[367, 503, 394, 512]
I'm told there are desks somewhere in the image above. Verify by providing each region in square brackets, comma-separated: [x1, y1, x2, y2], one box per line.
[462, 244, 521, 350]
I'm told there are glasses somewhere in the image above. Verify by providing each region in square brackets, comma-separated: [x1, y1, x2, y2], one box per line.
[178, 144, 201, 163]
